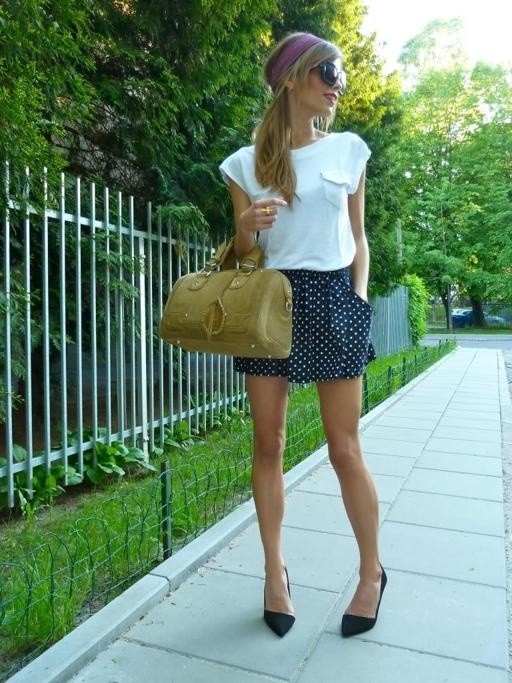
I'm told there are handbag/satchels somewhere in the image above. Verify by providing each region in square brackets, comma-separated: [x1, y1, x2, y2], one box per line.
[155, 235, 294, 362]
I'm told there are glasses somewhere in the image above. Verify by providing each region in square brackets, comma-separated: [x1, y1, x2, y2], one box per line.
[309, 60, 348, 97]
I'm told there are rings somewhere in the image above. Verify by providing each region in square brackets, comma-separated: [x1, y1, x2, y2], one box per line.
[261, 206, 273, 216]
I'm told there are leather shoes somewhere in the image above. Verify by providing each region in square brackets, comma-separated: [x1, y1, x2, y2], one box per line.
[340, 564, 389, 640]
[259, 567, 298, 638]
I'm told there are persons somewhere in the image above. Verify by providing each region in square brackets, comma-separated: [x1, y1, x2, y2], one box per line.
[218, 32, 387, 640]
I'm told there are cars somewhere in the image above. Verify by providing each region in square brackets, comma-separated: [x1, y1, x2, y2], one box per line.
[450, 307, 505, 329]
